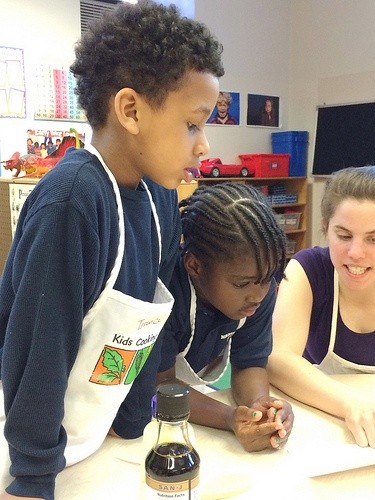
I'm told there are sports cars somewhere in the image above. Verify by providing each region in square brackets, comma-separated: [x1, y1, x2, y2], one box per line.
[199, 158, 254, 178]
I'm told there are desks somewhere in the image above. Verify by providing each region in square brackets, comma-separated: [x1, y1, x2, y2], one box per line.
[53, 373, 375, 500]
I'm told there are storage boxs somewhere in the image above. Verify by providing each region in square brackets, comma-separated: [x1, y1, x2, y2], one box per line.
[239, 154, 291, 178]
[271, 131, 309, 178]
[285, 239, 296, 254]
[277, 211, 302, 230]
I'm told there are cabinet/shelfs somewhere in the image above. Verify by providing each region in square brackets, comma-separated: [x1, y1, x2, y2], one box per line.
[176, 179, 198, 245]
[195, 177, 307, 258]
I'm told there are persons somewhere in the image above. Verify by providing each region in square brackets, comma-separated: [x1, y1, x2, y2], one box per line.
[209, 91, 237, 125]
[0, 0, 225, 500]
[256, 97, 276, 126]
[156, 166, 375, 453]
[27, 130, 61, 158]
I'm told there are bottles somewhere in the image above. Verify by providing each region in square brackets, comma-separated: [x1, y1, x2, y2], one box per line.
[143, 394, 196, 452]
[145, 383, 200, 500]
